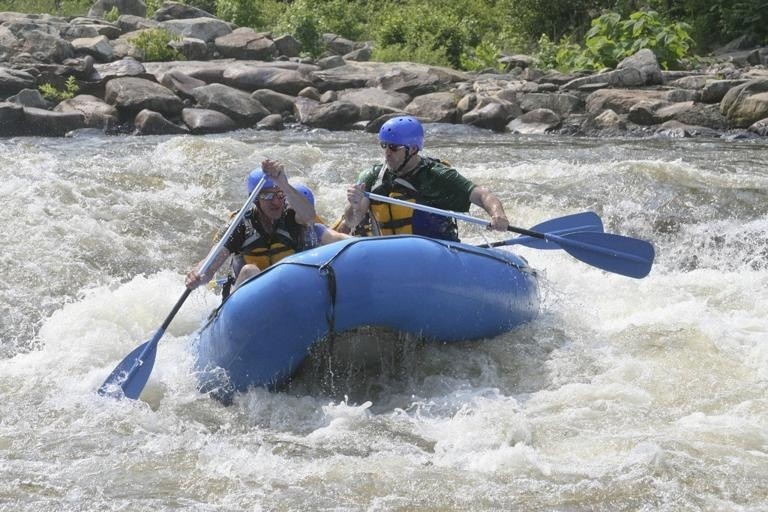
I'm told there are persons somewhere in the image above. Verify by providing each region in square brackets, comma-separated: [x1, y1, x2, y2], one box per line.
[344, 117, 510, 243]
[184, 160, 316, 293]
[293, 187, 356, 250]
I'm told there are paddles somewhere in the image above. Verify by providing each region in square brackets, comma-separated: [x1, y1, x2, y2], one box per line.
[360, 189, 654, 279]
[479, 212, 603, 251]
[96, 162, 280, 402]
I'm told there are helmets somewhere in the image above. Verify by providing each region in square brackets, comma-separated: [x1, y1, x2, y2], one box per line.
[378, 116, 424, 151]
[247, 167, 315, 207]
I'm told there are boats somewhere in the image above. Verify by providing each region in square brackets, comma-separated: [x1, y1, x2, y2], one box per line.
[196, 234, 542, 406]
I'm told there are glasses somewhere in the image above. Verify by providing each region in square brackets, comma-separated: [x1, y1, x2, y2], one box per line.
[380, 141, 409, 152]
